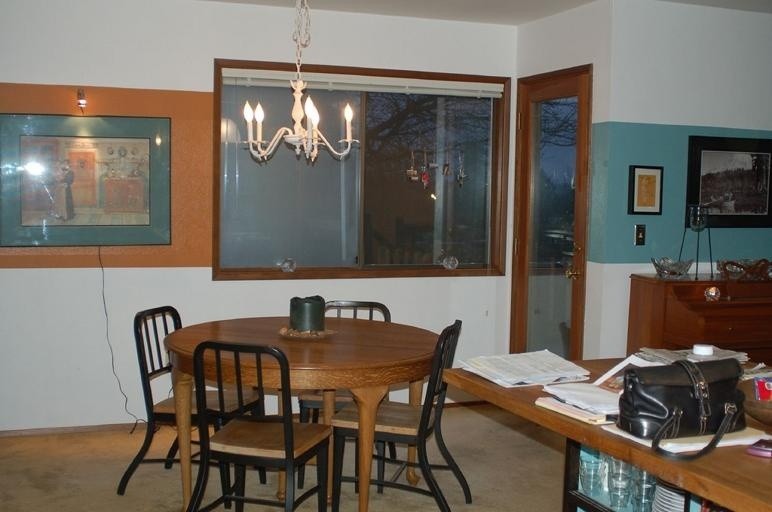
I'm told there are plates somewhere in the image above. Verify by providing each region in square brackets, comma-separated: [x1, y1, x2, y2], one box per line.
[652, 484, 685, 511]
[278, 326, 338, 338]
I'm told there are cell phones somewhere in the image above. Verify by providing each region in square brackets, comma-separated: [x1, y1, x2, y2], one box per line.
[746, 438, 772, 458]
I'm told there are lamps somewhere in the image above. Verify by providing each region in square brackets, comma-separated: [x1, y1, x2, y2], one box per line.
[77, 88, 87, 108]
[243, 0, 359, 162]
[678, 203, 713, 280]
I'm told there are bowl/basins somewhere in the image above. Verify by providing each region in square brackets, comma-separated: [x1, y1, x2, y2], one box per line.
[717, 258, 772, 281]
[651, 256, 694, 280]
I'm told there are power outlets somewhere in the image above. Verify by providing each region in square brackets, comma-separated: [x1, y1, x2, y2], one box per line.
[634, 224, 646, 247]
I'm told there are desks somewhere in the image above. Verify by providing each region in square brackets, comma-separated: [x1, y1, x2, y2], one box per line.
[163, 315, 447, 512]
[442, 352, 772, 511]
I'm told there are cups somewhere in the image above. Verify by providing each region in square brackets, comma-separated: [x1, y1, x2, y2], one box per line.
[289, 296, 325, 333]
[581, 447, 655, 511]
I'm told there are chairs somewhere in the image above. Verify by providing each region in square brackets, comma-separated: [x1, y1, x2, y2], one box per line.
[117, 304, 265, 497]
[185, 339, 332, 512]
[297, 300, 395, 493]
[330, 319, 472, 511]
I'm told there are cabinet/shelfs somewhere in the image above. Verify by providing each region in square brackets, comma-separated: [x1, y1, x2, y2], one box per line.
[627, 274, 772, 368]
[104, 177, 145, 215]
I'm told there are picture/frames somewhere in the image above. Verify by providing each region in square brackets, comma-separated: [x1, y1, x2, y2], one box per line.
[0, 113, 171, 248]
[628, 165, 664, 215]
[684, 135, 772, 229]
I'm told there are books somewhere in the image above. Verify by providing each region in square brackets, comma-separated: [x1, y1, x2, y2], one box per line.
[456, 345, 772, 453]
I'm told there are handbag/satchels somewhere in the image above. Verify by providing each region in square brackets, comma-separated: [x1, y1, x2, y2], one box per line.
[616, 358, 746, 439]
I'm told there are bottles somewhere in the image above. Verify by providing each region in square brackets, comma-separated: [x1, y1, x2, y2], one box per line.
[441, 256, 459, 271]
[280, 258, 297, 273]
